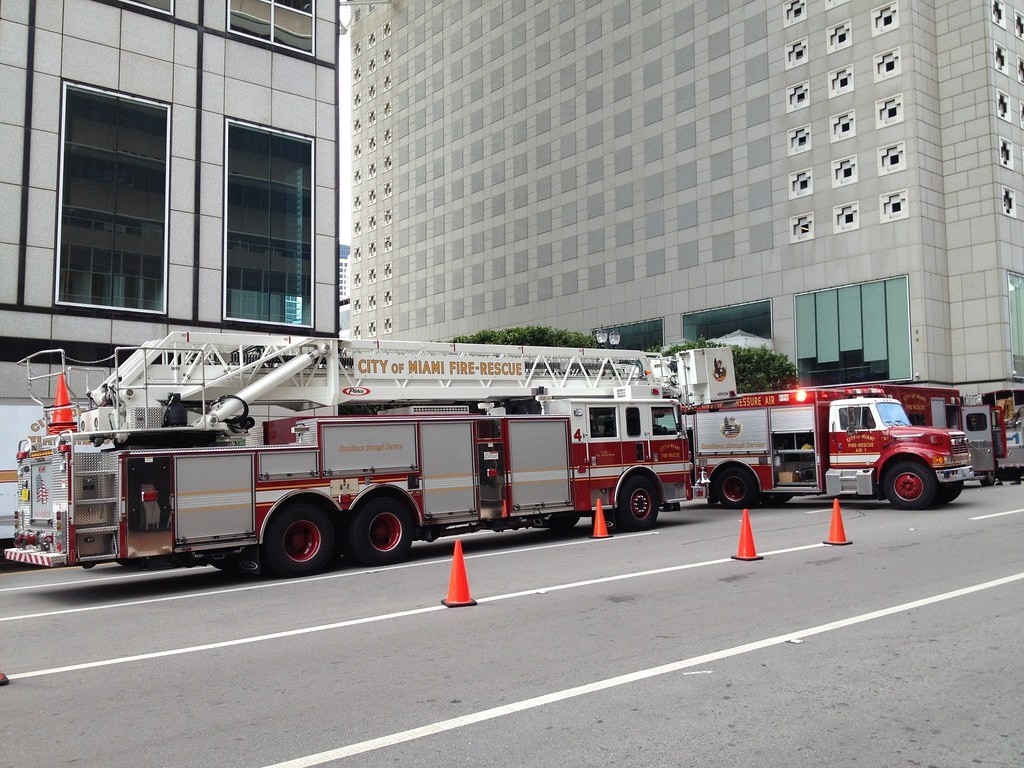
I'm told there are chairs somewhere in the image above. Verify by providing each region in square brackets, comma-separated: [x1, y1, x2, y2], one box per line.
[603, 413, 640, 435]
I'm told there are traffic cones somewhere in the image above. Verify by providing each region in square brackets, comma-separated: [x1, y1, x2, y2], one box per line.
[47, 374, 78, 435]
[441, 540, 477, 608]
[588, 499, 613, 538]
[823, 499, 852, 545]
[731, 509, 764, 561]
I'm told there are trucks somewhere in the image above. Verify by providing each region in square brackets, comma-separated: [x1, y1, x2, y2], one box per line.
[966, 397, 1024, 487]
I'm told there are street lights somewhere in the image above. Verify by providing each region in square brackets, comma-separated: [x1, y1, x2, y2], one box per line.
[596, 324, 620, 349]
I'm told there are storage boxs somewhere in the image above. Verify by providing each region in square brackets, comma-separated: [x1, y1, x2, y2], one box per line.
[779, 471, 801, 482]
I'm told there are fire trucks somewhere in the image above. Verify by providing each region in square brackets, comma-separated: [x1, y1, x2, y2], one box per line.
[845, 385, 1007, 471]
[5, 331, 743, 577]
[691, 388, 973, 511]
